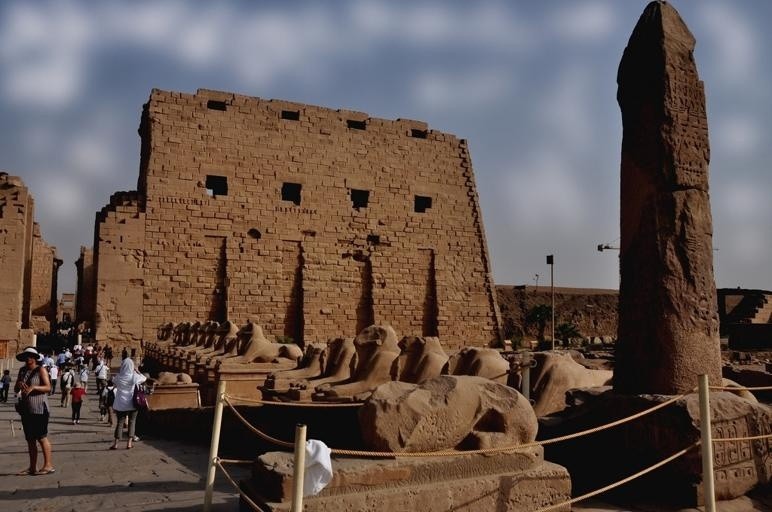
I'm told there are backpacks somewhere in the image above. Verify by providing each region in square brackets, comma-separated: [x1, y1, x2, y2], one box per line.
[107, 387, 115, 405]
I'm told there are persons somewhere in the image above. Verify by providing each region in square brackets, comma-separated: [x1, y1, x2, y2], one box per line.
[106, 357, 147, 450]
[14, 345, 57, 476]
[2, 321, 148, 427]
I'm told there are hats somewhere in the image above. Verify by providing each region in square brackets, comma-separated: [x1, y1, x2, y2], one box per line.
[16, 346, 40, 361]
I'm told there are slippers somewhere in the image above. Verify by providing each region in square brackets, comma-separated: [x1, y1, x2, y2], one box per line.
[110, 445, 117, 449]
[128, 444, 134, 449]
[17, 468, 38, 475]
[35, 467, 55, 475]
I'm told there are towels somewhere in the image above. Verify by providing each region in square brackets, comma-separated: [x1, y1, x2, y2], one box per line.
[298, 435, 337, 500]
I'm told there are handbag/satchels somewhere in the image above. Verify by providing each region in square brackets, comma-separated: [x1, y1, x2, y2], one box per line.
[134, 391, 148, 409]
